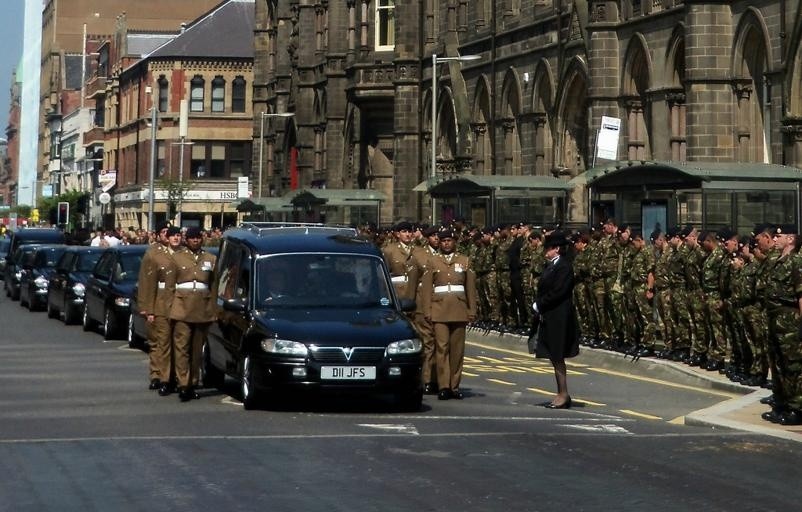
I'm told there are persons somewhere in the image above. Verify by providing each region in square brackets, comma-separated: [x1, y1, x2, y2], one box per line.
[164, 228, 220, 402]
[135, 220, 173, 390]
[144, 226, 185, 397]
[407, 225, 443, 394]
[528, 229, 581, 409]
[422, 230, 479, 401]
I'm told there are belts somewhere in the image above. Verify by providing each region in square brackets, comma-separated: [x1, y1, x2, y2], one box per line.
[157, 280, 166, 288]
[391, 275, 409, 283]
[434, 284, 465, 293]
[176, 280, 208, 292]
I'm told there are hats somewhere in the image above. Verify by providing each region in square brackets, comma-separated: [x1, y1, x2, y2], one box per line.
[436, 229, 459, 240]
[421, 225, 444, 236]
[697, 230, 710, 245]
[618, 222, 630, 235]
[628, 233, 637, 244]
[591, 215, 609, 232]
[664, 228, 678, 241]
[737, 234, 759, 253]
[650, 230, 661, 242]
[540, 226, 555, 234]
[451, 215, 542, 241]
[396, 222, 412, 232]
[570, 229, 585, 248]
[184, 227, 202, 239]
[715, 226, 738, 243]
[678, 224, 694, 241]
[771, 223, 799, 234]
[750, 222, 772, 239]
[726, 251, 744, 259]
[154, 220, 182, 237]
[540, 233, 570, 247]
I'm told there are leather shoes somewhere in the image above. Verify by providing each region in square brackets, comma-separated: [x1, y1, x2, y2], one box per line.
[423, 381, 434, 394]
[719, 367, 773, 390]
[759, 392, 802, 426]
[467, 320, 534, 336]
[577, 336, 656, 358]
[656, 348, 726, 372]
[438, 387, 465, 400]
[149, 378, 201, 402]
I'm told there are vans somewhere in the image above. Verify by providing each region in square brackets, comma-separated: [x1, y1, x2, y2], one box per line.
[197, 219, 427, 410]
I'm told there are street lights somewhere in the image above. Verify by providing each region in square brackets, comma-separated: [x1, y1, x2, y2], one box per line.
[32, 178, 47, 227]
[16, 185, 29, 205]
[138, 82, 160, 243]
[81, 11, 101, 107]
[258, 110, 298, 204]
[431, 52, 484, 227]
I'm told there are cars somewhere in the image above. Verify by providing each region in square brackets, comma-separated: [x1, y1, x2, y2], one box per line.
[0, 226, 220, 348]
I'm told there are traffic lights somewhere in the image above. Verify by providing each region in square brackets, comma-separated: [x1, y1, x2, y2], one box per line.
[59, 202, 69, 223]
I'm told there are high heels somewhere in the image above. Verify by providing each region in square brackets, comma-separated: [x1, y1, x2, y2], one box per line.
[545, 395, 573, 410]
